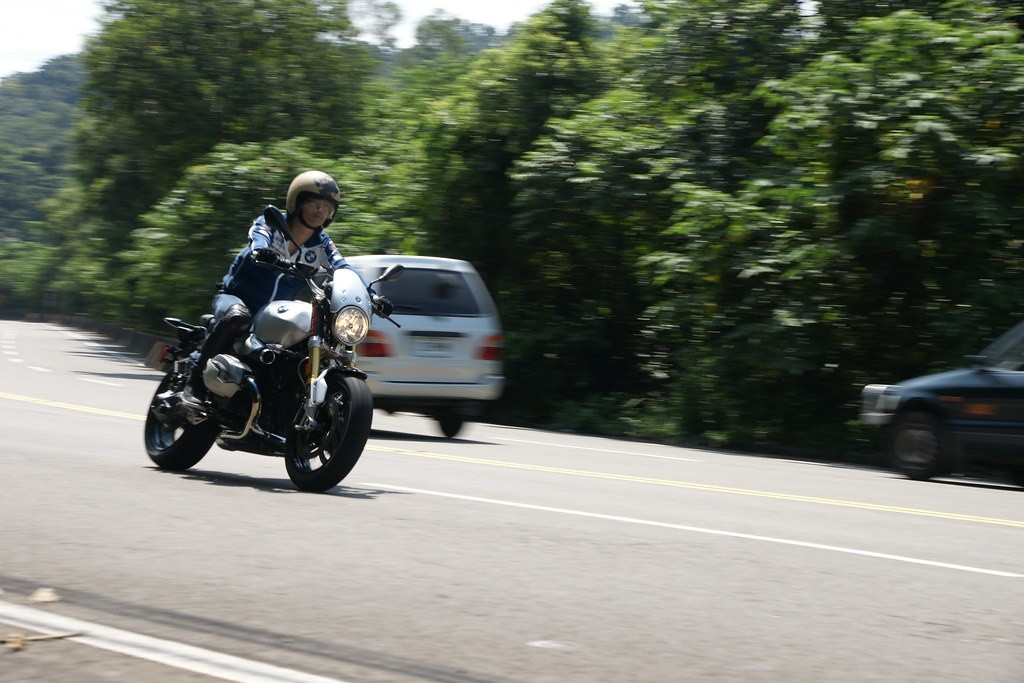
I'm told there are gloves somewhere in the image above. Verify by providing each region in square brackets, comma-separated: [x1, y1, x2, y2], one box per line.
[252, 248, 280, 265]
[370, 293, 394, 318]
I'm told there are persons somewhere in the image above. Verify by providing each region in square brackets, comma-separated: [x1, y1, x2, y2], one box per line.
[180, 171, 395, 410]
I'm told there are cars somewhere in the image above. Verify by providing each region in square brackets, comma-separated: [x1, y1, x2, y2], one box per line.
[859, 317, 1024, 482]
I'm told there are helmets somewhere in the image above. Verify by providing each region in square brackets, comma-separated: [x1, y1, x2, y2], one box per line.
[286, 171, 340, 228]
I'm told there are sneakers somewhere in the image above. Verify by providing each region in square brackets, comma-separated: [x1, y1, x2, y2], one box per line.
[181, 378, 211, 411]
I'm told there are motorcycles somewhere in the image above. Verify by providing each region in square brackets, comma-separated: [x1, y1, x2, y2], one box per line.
[143, 204, 406, 494]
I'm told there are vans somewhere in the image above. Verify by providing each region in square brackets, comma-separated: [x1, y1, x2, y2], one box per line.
[327, 254, 509, 438]
